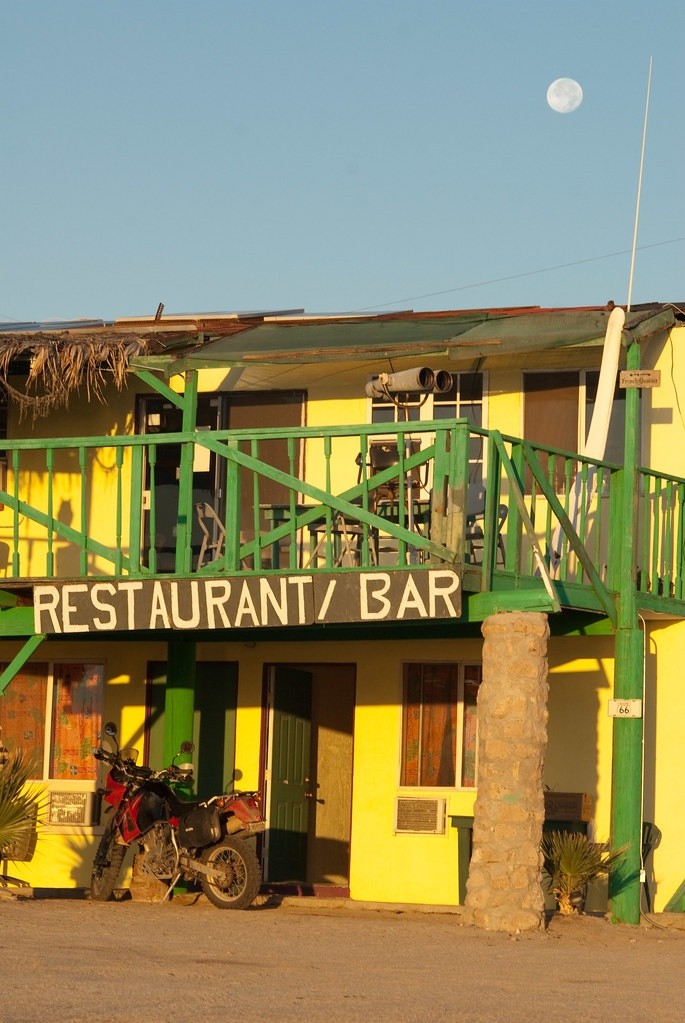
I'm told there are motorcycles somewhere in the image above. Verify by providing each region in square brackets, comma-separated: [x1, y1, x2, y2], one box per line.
[90, 721, 268, 910]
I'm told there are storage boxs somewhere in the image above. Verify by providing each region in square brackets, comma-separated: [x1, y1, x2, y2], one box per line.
[543, 791, 592, 822]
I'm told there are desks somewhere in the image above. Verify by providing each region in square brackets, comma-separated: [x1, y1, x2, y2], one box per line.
[448, 814, 588, 905]
[255, 504, 361, 567]
[378, 499, 428, 565]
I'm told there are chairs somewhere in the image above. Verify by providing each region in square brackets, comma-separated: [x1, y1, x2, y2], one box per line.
[194, 501, 253, 572]
[465, 503, 507, 569]
[298, 498, 378, 568]
[0, 796, 38, 887]
[583, 819, 662, 914]
